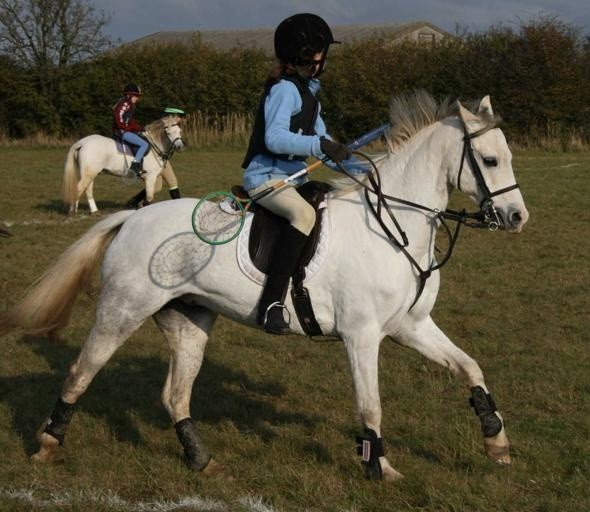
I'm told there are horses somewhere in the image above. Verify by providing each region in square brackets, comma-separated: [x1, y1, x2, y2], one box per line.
[61, 110, 187, 218]
[3, 90, 531, 484]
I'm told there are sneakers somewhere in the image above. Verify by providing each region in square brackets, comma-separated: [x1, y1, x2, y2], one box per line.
[130, 161, 146, 173]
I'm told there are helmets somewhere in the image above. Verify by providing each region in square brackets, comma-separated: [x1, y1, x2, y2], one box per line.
[122, 83, 143, 96]
[274, 14, 342, 63]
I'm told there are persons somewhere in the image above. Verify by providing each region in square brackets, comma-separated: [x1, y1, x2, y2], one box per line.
[242, 13, 372, 336]
[112, 83, 149, 173]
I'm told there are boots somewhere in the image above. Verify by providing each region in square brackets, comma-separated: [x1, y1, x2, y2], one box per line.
[256, 224, 309, 333]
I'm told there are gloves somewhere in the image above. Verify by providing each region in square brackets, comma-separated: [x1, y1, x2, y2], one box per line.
[319, 135, 351, 162]
